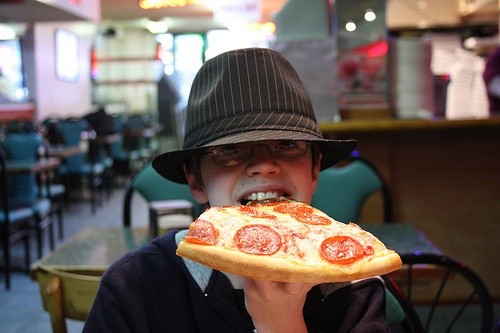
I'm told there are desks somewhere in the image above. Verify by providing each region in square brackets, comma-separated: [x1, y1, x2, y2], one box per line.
[30, 225, 453, 333]
[5, 157, 60, 272]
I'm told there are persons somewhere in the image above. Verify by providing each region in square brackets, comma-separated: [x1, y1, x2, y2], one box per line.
[82, 47, 386, 333]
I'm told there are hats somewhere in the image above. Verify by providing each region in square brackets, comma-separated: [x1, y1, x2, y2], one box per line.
[152, 48, 361, 184]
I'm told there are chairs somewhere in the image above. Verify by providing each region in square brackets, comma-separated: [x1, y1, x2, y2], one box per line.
[313, 152, 494, 333]
[0, 114, 207, 333]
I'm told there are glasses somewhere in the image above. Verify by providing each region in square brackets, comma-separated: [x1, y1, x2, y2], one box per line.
[203, 138, 311, 168]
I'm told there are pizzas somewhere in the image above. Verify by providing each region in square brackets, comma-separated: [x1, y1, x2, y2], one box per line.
[176, 195, 402, 284]
